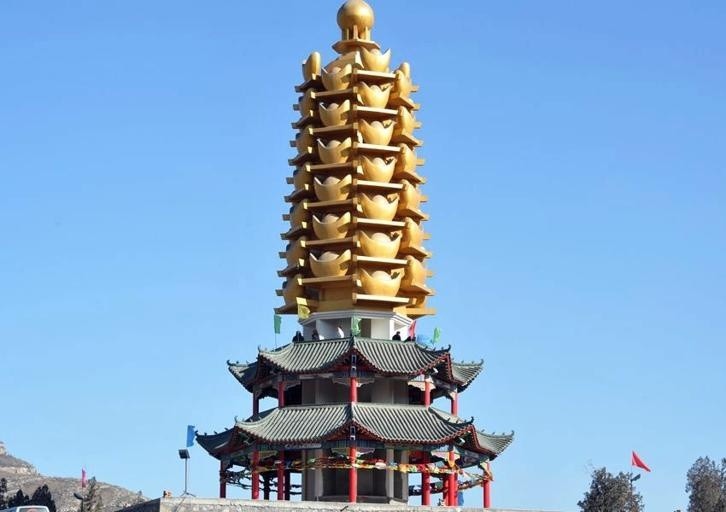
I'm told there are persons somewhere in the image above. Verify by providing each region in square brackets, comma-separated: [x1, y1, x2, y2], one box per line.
[335, 325, 343, 337]
[392, 330, 401, 340]
[291, 330, 304, 342]
[311, 329, 320, 341]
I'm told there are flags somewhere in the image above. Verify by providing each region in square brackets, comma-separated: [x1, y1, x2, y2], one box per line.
[273, 315, 282, 333]
[433, 328, 440, 343]
[407, 321, 416, 340]
[81, 468, 87, 488]
[185, 425, 196, 448]
[630, 451, 650, 472]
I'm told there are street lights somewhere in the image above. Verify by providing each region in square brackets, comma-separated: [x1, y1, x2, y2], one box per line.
[631, 474, 640, 508]
[179, 450, 196, 497]
[74, 493, 84, 512]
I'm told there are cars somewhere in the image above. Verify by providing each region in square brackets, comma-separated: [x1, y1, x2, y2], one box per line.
[6, 505, 50, 512]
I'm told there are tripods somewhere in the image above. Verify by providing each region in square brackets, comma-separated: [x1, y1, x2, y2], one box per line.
[180, 459, 196, 497]
